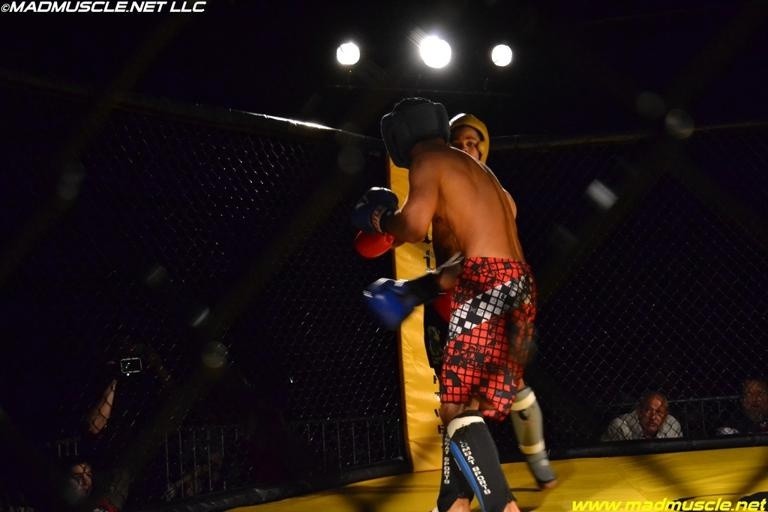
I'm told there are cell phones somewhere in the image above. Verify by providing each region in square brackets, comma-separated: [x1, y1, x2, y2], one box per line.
[120, 357, 143, 374]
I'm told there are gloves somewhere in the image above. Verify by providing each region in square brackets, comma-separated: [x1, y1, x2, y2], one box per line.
[433, 291, 452, 324]
[353, 231, 396, 258]
[362, 278, 418, 333]
[351, 187, 399, 233]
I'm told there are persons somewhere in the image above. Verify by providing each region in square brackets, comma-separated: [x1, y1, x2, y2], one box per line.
[604, 393, 683, 444]
[1, 312, 269, 511]
[340, 114, 558, 490]
[715, 378, 767, 436]
[355, 97, 539, 512]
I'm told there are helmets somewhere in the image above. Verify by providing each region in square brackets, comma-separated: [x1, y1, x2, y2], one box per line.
[381, 102, 450, 168]
[451, 111, 490, 164]
